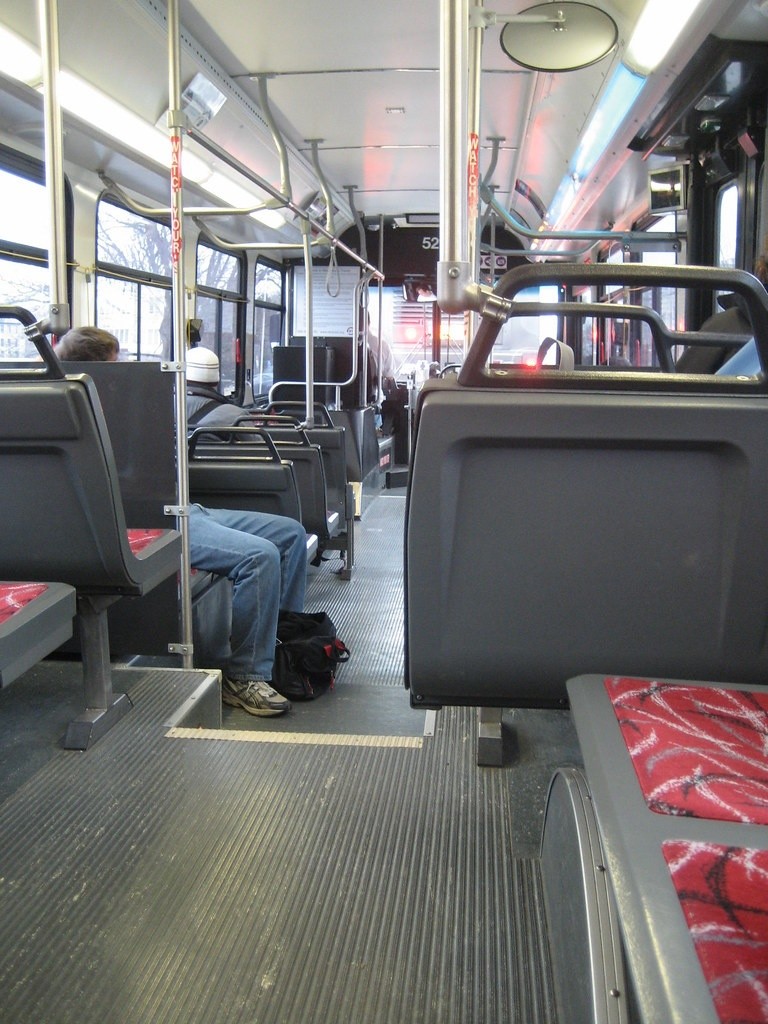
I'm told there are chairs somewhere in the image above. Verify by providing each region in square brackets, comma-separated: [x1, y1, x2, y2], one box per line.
[0, 263, 768, 1024]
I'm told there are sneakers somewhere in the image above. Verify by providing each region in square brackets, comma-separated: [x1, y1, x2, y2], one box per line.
[223, 676, 292, 717]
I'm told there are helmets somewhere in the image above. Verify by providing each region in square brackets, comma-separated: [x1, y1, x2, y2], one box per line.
[185, 347, 219, 382]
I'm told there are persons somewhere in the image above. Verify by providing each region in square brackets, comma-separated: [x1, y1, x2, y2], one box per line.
[673, 231, 768, 374]
[54, 326, 310, 716]
[360, 306, 396, 431]
[416, 285, 437, 302]
[173, 346, 264, 443]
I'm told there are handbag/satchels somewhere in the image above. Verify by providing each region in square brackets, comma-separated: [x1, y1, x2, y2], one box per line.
[269, 608, 351, 702]
